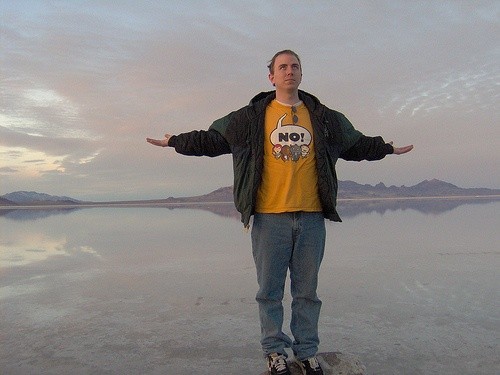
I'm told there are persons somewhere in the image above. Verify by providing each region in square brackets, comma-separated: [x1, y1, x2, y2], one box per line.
[143, 50, 417, 375]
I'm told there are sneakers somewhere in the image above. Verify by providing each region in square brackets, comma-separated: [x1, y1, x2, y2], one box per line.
[265, 353, 291, 375]
[294, 354, 324, 375]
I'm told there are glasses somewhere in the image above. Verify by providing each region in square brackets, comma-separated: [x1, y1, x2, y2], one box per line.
[291, 105, 299, 125]
